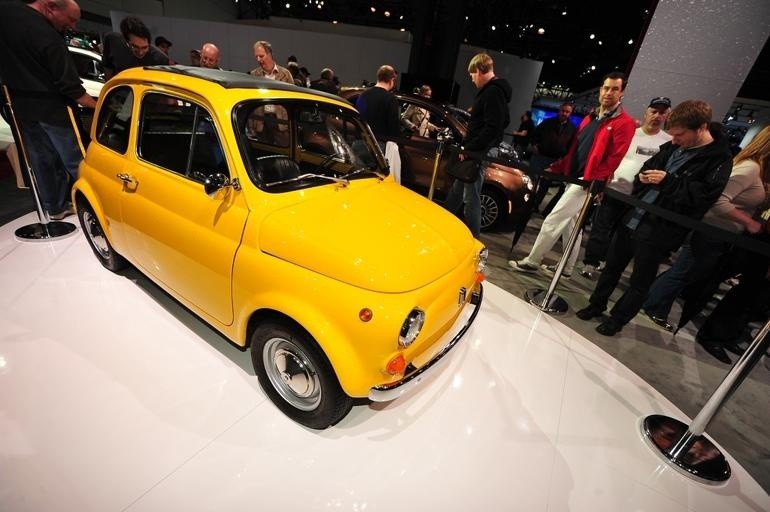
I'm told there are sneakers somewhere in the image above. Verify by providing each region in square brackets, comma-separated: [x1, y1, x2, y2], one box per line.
[47, 202, 75, 221]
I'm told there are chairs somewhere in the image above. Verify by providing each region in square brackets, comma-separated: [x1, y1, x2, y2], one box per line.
[257, 153, 301, 184]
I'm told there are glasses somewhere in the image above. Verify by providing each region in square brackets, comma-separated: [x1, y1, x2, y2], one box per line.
[202, 59, 215, 66]
[125, 40, 152, 51]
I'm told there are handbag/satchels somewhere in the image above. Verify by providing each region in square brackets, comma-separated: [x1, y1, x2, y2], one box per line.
[443, 155, 480, 184]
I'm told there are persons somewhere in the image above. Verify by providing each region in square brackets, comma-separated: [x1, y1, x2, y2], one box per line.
[1, 2, 445, 225]
[439, 52, 513, 242]
[506, 69, 769, 364]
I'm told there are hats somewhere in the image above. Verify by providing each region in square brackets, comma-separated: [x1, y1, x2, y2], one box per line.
[650, 97, 671, 110]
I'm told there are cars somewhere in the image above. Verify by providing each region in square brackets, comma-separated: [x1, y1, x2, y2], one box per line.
[439, 103, 520, 164]
[301, 86, 534, 233]
[66, 46, 107, 108]
[70, 63, 489, 428]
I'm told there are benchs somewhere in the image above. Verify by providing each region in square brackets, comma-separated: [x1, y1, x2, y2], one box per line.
[139, 128, 241, 180]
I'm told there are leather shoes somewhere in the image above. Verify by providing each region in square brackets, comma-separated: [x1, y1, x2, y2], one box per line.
[509, 260, 745, 364]
